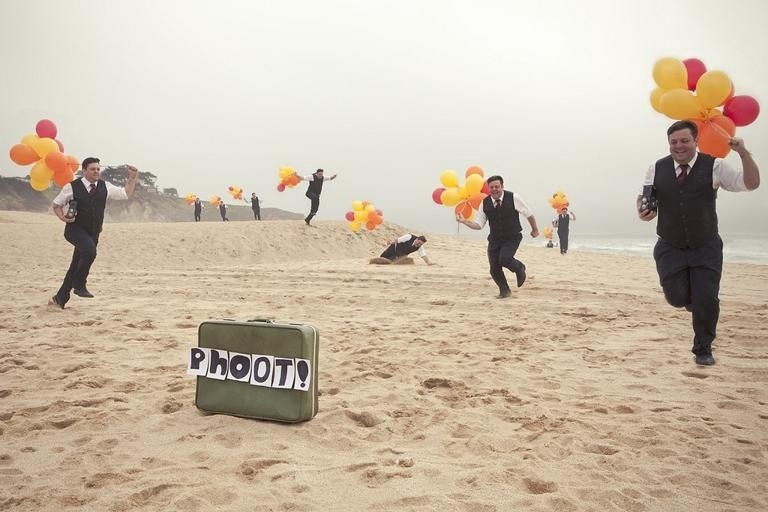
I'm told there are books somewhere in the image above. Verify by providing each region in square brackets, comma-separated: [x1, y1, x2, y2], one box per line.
[226, 183, 244, 201]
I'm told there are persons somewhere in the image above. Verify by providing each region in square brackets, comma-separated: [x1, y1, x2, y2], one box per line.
[244, 193, 263, 220]
[544, 239, 558, 249]
[380, 233, 436, 265]
[636, 119, 760, 365]
[217, 201, 229, 221]
[457, 175, 539, 299]
[295, 168, 337, 226]
[552, 207, 576, 254]
[50, 158, 138, 311]
[190, 197, 205, 221]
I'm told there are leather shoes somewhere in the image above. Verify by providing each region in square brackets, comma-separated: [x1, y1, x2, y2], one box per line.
[516, 264, 526, 287]
[53, 295, 64, 309]
[73, 286, 94, 298]
[696, 352, 715, 365]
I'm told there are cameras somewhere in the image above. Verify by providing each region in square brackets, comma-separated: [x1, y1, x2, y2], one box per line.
[66, 201, 78, 219]
[640, 185, 657, 213]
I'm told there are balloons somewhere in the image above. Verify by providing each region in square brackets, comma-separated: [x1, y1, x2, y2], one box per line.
[276, 165, 302, 192]
[650, 55, 759, 159]
[208, 194, 221, 208]
[431, 164, 490, 233]
[547, 189, 569, 214]
[184, 192, 196, 206]
[345, 198, 392, 239]
[542, 226, 554, 239]
[9, 120, 136, 192]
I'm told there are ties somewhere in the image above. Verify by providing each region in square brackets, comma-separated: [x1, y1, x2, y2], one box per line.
[495, 199, 501, 210]
[89, 183, 97, 194]
[677, 164, 689, 183]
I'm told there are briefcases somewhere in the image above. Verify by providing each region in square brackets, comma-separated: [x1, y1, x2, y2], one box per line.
[195, 317, 322, 426]
